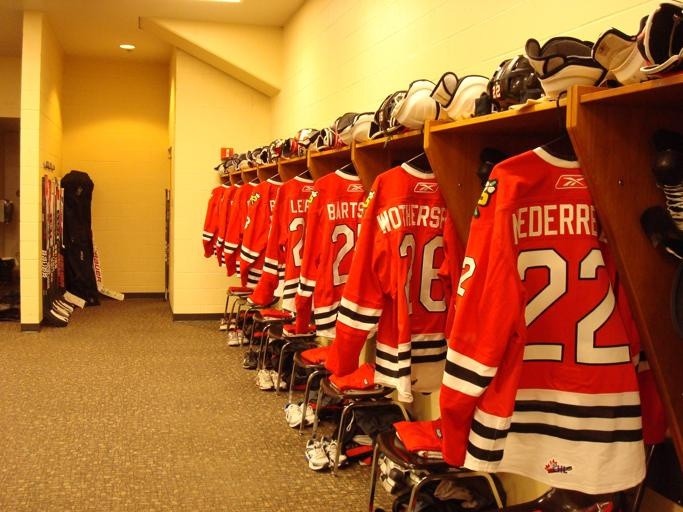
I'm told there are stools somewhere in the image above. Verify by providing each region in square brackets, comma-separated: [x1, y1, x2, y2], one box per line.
[312, 377, 415, 477]
[370, 426, 503, 511]
[290, 350, 385, 435]
[262, 322, 321, 396]
[240, 307, 289, 371]
[226, 298, 264, 337]
[225, 286, 257, 325]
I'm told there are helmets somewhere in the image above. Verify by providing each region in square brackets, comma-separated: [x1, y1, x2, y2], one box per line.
[215, 112, 374, 175]
[369, 71, 488, 139]
[486, 0, 683, 112]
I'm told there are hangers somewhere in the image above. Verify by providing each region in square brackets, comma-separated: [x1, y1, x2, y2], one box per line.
[235, 179, 242, 185]
[269, 173, 278, 179]
[223, 180, 229, 185]
[540, 89, 569, 146]
[406, 152, 425, 163]
[339, 162, 352, 171]
[250, 176, 258, 183]
[297, 169, 307, 177]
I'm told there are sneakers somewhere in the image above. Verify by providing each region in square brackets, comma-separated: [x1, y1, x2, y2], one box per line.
[219, 318, 249, 345]
[305, 434, 349, 471]
[243, 349, 273, 370]
[253, 369, 287, 392]
[282, 401, 321, 429]
